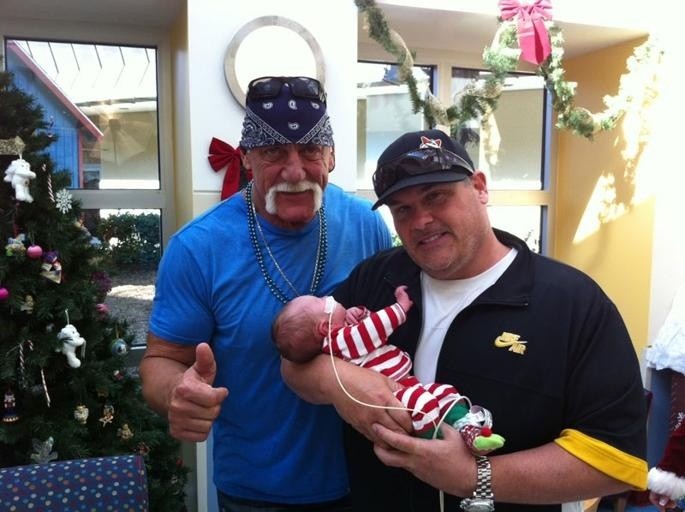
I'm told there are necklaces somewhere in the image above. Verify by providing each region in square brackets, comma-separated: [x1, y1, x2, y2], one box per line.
[246, 179, 327, 306]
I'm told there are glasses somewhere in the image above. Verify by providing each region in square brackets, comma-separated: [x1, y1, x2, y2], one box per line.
[370, 148, 475, 196]
[247, 76, 327, 104]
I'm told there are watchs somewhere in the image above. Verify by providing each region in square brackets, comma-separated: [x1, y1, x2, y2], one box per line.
[460, 455, 495, 512]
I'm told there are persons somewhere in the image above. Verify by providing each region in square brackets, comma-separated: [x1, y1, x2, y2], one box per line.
[271, 284, 505, 456]
[648, 417, 685, 512]
[280, 128, 649, 512]
[139, 76, 393, 511]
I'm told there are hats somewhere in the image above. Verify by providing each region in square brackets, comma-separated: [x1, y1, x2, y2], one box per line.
[240, 78, 333, 145]
[372, 130, 473, 212]
